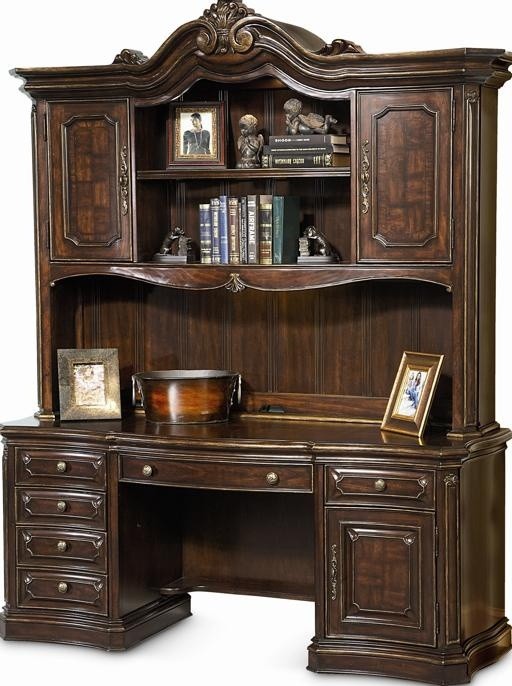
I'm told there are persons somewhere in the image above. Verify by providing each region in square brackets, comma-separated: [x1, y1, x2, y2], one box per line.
[183, 113, 212, 155]
[304, 226, 331, 256]
[237, 115, 263, 166]
[283, 98, 337, 135]
[405, 372, 421, 390]
[161, 225, 182, 256]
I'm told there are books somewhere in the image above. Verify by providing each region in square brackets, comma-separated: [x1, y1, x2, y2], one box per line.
[199, 195, 300, 266]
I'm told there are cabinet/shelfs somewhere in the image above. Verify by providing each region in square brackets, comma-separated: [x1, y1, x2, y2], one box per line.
[0, 0, 512, 686]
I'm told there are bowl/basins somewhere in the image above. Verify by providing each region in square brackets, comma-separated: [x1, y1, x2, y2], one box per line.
[129, 368, 243, 428]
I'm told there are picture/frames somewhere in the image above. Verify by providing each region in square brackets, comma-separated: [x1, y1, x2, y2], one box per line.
[56, 343, 120, 422]
[379, 346, 445, 446]
[164, 98, 227, 172]
